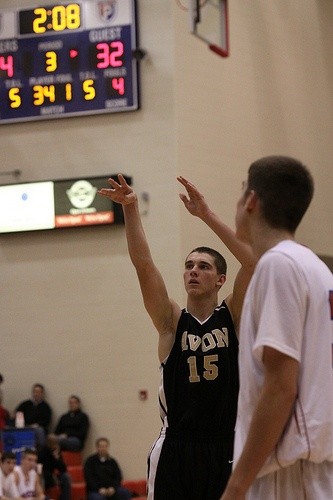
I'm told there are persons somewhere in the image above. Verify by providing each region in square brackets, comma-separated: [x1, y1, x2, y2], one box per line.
[38, 434, 73, 500]
[14, 383, 54, 456]
[84, 438, 123, 500]
[0, 451, 24, 500]
[97, 172, 256, 500]
[221, 154, 333, 500]
[12, 448, 46, 500]
[49, 396, 90, 451]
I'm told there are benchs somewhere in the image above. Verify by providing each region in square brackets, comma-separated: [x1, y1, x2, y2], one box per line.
[48, 452, 148, 500]
